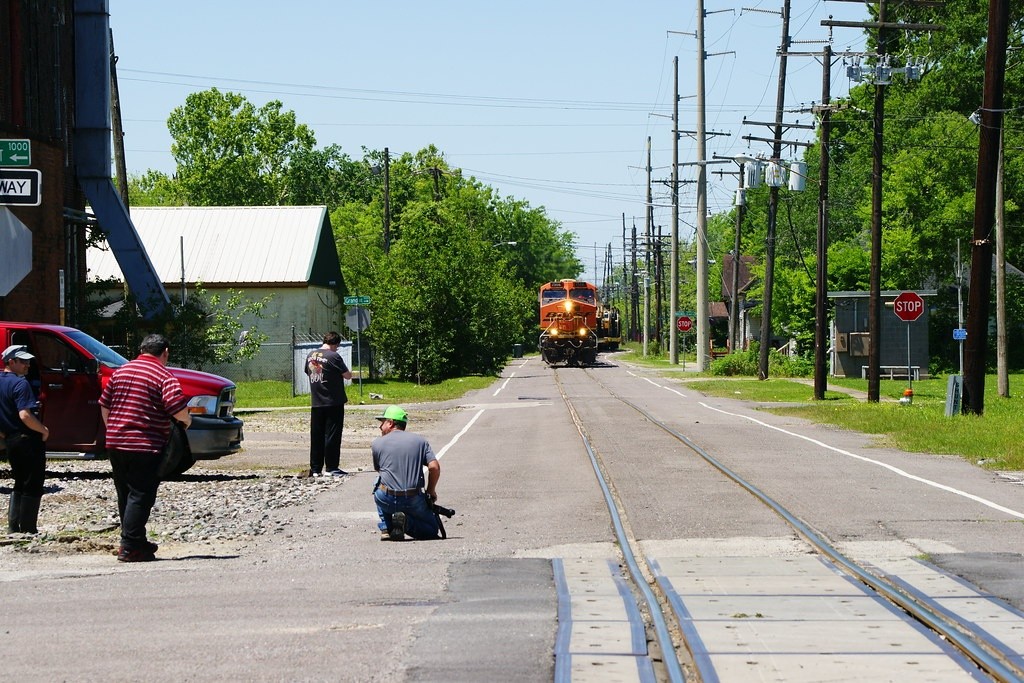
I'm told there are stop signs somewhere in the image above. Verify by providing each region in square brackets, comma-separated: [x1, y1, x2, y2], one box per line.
[893, 292, 926, 321]
[677, 316, 693, 332]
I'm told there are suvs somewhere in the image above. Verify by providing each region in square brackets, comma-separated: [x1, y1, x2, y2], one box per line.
[0, 321, 244, 480]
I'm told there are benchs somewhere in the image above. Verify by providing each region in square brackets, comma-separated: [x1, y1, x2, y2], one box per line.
[861, 366, 921, 381]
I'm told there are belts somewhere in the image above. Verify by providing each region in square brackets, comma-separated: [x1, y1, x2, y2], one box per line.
[381, 484, 418, 496]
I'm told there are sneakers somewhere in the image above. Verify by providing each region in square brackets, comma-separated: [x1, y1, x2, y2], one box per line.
[325, 467, 348, 476]
[311, 470, 323, 476]
[117, 544, 155, 562]
[143, 541, 158, 552]
[381, 529, 391, 540]
[391, 511, 406, 541]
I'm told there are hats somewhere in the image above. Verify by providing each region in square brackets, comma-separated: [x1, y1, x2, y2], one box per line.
[375, 404, 408, 423]
[1, 345, 36, 363]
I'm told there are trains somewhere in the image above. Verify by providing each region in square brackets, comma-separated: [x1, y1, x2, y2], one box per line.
[538, 278, 622, 368]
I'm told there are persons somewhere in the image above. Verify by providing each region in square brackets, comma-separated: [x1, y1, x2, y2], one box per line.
[0, 345, 49, 535]
[304, 332, 352, 476]
[98, 334, 192, 562]
[371, 405, 440, 541]
